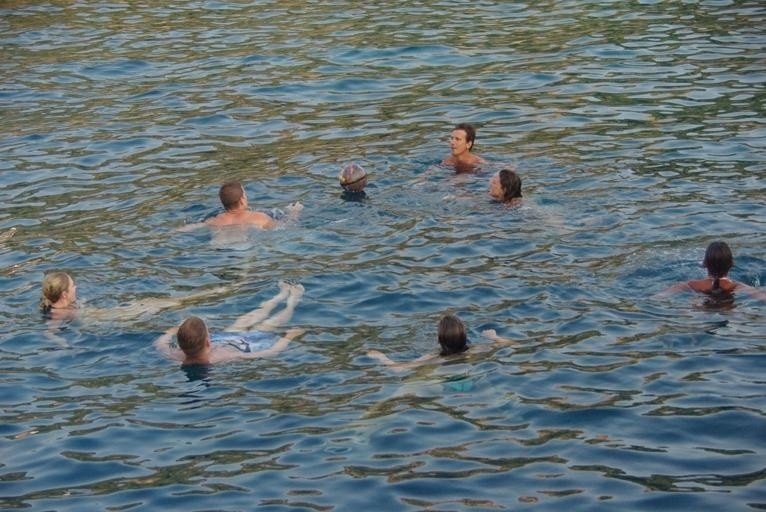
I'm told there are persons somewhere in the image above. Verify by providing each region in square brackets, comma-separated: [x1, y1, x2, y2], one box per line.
[366, 315, 518, 374]
[154, 278, 306, 366]
[37, 270, 243, 349]
[441, 167, 540, 210]
[177, 182, 303, 247]
[405, 121, 491, 189]
[651, 242, 764, 304]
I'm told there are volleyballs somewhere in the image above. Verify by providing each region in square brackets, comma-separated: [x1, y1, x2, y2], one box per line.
[339, 164, 366, 192]
